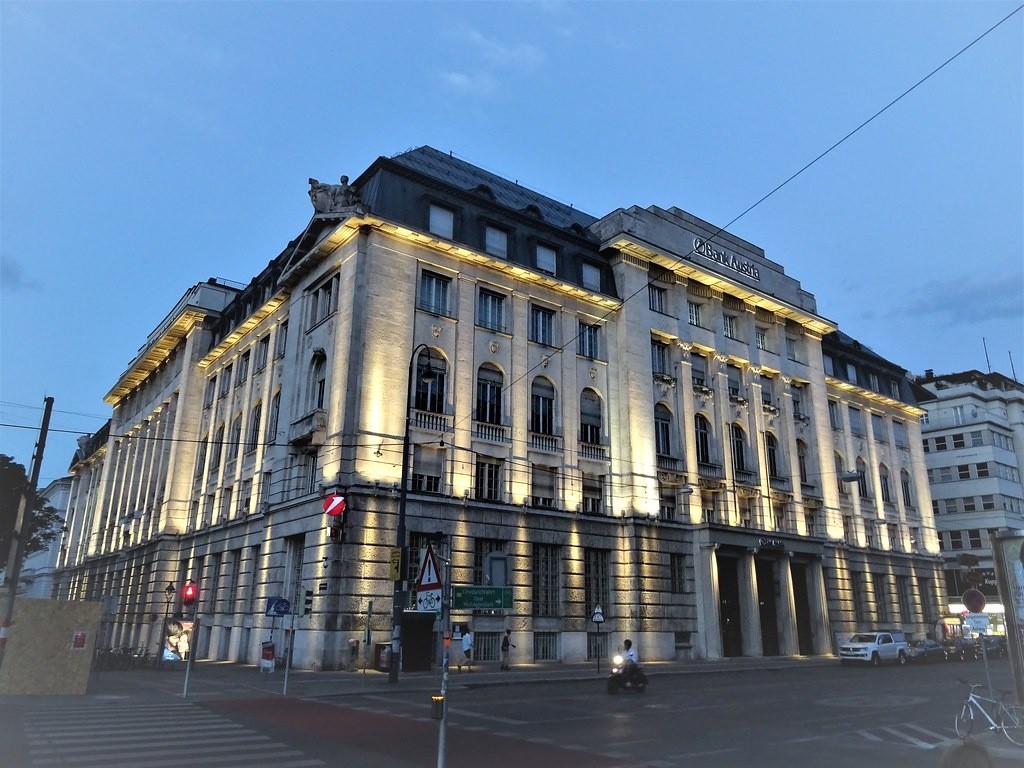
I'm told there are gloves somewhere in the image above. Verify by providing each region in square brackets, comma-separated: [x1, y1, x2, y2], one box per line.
[514, 646, 516, 647]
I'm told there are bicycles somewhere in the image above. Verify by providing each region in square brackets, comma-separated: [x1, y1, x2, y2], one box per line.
[97, 645, 151, 672]
[955, 678, 1024, 746]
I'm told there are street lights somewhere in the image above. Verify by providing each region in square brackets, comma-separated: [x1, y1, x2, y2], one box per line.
[156, 581, 176, 671]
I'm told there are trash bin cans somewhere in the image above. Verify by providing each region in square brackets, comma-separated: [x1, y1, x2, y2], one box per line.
[375, 641, 402, 683]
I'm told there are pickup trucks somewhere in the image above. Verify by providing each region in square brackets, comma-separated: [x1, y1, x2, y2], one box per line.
[838, 632, 909, 668]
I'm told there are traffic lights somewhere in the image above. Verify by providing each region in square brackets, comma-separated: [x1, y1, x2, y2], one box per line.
[184, 585, 195, 605]
[330, 527, 339, 541]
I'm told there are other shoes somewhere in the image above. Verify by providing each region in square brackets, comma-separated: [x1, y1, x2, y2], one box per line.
[458, 664, 460, 672]
[467, 670, 474, 672]
[501, 665, 510, 670]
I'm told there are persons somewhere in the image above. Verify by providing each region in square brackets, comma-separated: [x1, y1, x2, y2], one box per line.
[609, 639, 638, 687]
[499, 629, 516, 670]
[457, 626, 475, 673]
[164, 620, 190, 663]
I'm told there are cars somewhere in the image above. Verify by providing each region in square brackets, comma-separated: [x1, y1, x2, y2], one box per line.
[944, 634, 1007, 661]
[908, 640, 948, 664]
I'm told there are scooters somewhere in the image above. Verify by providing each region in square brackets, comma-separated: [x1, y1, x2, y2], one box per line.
[607, 652, 648, 693]
